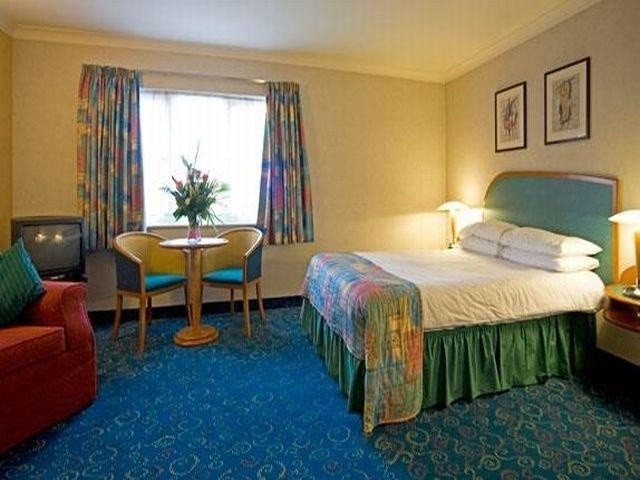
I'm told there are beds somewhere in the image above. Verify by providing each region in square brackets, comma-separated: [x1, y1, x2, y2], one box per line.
[297, 170, 620, 437]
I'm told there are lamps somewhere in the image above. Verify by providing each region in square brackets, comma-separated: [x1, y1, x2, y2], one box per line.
[608, 208, 640, 299]
[438, 201, 469, 249]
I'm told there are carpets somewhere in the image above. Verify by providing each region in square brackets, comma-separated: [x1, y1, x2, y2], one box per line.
[1, 307, 640, 480]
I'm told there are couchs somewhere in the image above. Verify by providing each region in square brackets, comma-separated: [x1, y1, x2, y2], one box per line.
[0, 282, 98, 453]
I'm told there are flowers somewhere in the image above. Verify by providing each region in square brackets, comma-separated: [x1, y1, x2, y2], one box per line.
[159, 137, 230, 237]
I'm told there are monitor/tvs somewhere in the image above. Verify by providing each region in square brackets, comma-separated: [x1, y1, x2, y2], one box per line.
[11, 216, 86, 277]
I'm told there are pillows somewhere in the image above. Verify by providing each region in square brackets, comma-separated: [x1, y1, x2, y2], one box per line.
[0, 237, 46, 327]
[499, 226, 598, 259]
[502, 251, 601, 273]
[460, 235, 508, 257]
[461, 219, 520, 244]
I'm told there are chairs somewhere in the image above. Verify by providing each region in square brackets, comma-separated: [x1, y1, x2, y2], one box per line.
[111, 232, 192, 354]
[195, 227, 266, 339]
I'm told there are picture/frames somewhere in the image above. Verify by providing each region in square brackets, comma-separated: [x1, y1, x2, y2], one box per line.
[543, 59, 590, 147]
[495, 79, 526, 152]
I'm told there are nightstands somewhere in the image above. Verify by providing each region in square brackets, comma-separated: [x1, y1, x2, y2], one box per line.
[594, 282, 640, 386]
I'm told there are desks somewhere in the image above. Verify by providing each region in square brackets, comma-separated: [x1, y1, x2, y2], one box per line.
[159, 237, 229, 347]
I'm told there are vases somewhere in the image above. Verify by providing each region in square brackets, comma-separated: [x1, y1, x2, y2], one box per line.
[187, 218, 202, 244]
